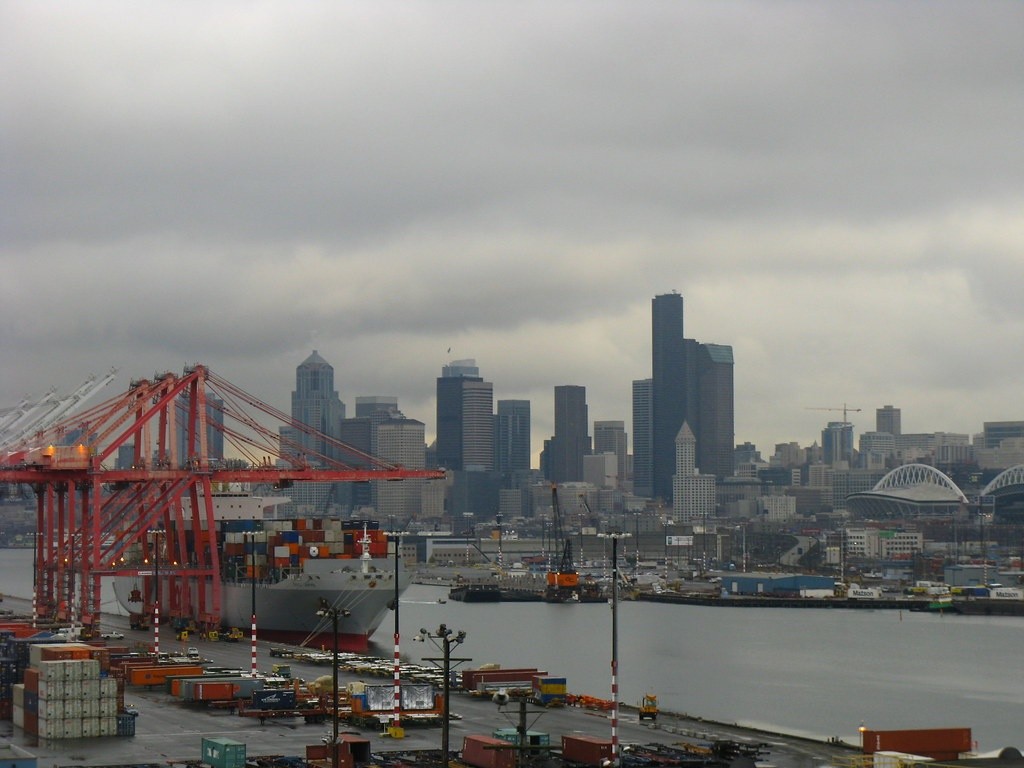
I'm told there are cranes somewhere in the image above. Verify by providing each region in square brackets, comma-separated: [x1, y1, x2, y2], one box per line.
[546, 478, 580, 590]
[808, 404, 861, 458]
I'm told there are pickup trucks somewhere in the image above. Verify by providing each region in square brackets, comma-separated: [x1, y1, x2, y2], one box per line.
[101, 631, 124, 640]
[187, 648, 199, 657]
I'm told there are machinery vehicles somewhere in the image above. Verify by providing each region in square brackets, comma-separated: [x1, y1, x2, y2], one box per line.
[639, 695, 659, 720]
[147, 530, 167, 658]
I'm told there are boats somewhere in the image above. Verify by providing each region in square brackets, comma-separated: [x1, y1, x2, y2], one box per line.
[111, 483, 419, 653]
[437, 600, 446, 604]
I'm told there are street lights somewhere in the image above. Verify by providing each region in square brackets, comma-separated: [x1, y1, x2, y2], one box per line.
[700, 512, 709, 574]
[601, 520, 607, 584]
[634, 512, 641, 578]
[462, 512, 474, 564]
[27, 532, 43, 628]
[242, 530, 263, 678]
[662, 522, 669, 580]
[736, 525, 746, 574]
[578, 514, 586, 574]
[597, 532, 632, 761]
[540, 513, 553, 573]
[984, 514, 991, 585]
